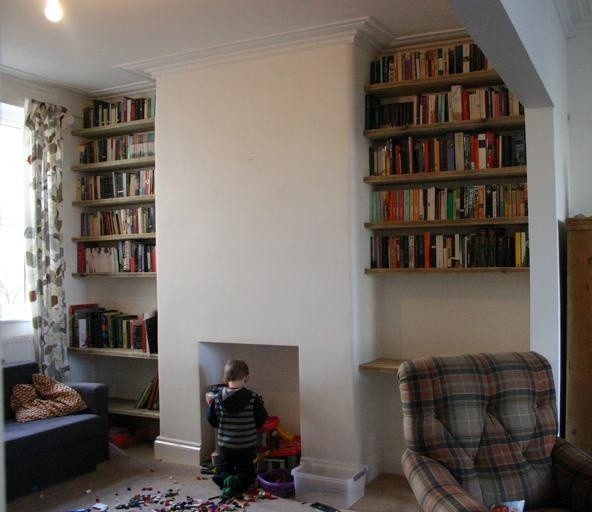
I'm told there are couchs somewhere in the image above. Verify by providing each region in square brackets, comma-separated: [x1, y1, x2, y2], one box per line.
[397, 348, 592, 512]
[0, 360, 109, 502]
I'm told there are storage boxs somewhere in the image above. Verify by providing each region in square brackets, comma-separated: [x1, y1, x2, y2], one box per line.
[291, 459, 368, 510]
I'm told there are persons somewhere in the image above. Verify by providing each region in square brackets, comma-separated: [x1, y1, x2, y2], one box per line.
[206, 360, 268, 497]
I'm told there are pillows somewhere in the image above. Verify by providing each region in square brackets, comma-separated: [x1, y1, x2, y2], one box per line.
[10, 373, 87, 423]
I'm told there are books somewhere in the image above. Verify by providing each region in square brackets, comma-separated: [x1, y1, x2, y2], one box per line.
[135, 373, 159, 410]
[69, 303, 158, 355]
[371, 231, 529, 269]
[77, 241, 155, 274]
[370, 86, 524, 130]
[79, 131, 155, 164]
[371, 182, 528, 223]
[83, 95, 156, 128]
[371, 44, 492, 84]
[369, 133, 526, 176]
[75, 170, 154, 200]
[81, 206, 155, 236]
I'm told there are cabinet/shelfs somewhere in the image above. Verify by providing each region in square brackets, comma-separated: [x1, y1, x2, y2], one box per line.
[361, 36, 530, 476]
[70, 99, 160, 432]
